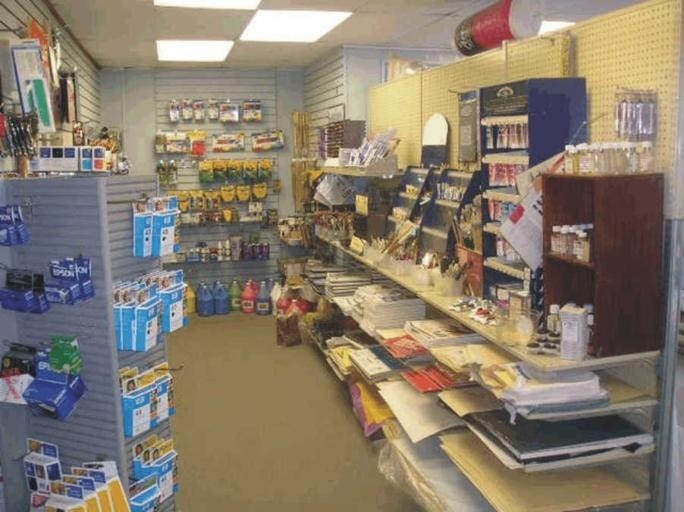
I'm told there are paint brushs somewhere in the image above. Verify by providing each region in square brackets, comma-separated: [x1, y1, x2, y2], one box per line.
[446, 193, 481, 256]
[371, 220, 417, 267]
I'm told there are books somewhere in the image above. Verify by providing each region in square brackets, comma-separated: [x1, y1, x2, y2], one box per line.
[300, 261, 655, 469]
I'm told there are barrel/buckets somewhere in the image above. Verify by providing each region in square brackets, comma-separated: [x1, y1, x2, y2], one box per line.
[183, 278, 274, 316]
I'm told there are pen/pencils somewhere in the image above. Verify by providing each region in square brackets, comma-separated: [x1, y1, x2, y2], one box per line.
[455, 262, 474, 282]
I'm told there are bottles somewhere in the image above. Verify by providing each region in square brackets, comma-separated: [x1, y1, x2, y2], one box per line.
[187, 239, 270, 263]
[544, 301, 593, 334]
[550, 220, 595, 264]
[563, 138, 655, 178]
